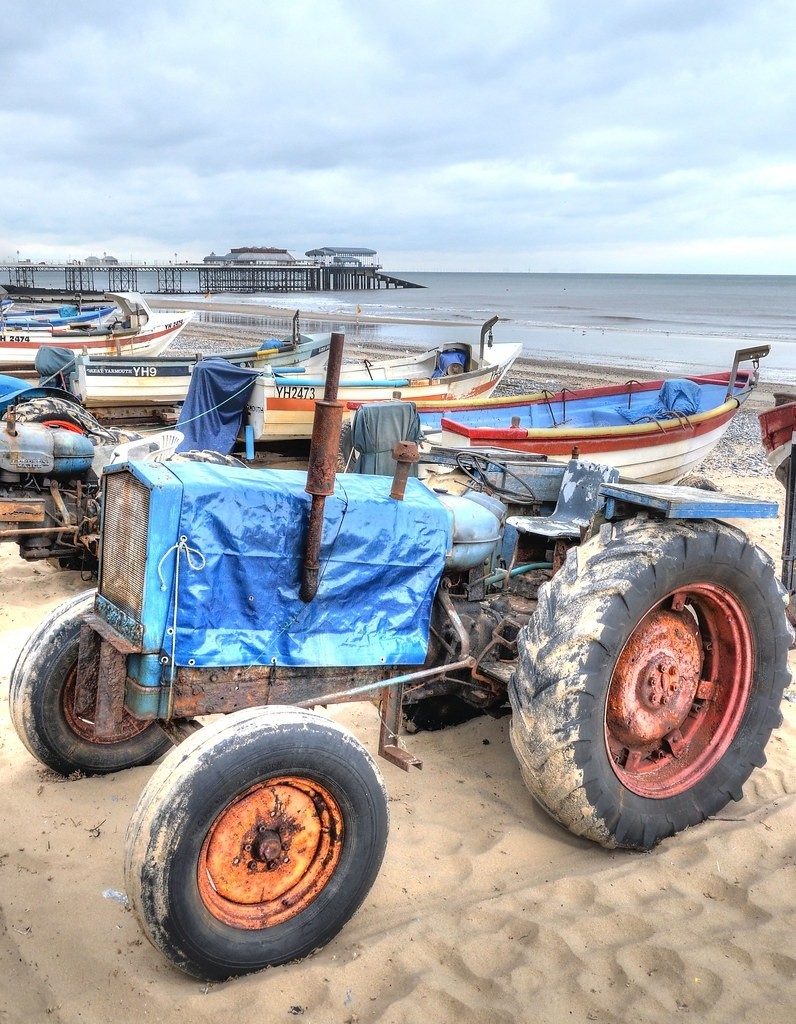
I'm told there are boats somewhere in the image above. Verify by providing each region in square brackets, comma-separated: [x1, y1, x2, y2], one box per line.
[0, 288, 796, 571]
